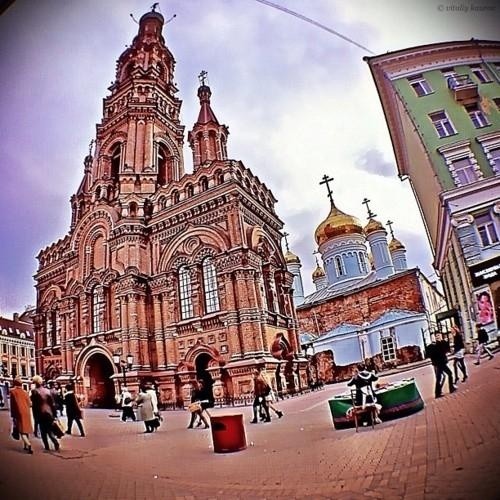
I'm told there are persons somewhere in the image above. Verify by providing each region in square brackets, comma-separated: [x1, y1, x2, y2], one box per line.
[427, 325, 468, 399]
[187, 379, 212, 428]
[347, 365, 383, 426]
[9, 374, 86, 455]
[248, 368, 284, 423]
[473, 324, 494, 365]
[307, 371, 326, 391]
[477, 292, 494, 324]
[115, 380, 163, 433]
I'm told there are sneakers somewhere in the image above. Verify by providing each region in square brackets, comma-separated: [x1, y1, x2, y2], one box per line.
[435, 388, 457, 398]
[22, 445, 59, 454]
[473, 354, 494, 365]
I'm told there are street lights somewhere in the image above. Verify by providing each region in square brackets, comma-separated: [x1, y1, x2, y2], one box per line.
[112, 354, 134, 386]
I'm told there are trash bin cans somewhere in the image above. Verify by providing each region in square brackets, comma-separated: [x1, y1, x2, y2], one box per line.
[211, 414, 246, 454]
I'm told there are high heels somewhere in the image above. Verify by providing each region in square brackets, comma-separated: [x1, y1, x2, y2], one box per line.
[188, 422, 209, 429]
[453, 376, 468, 384]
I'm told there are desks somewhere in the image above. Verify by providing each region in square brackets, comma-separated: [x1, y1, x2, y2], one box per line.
[328, 378, 424, 429]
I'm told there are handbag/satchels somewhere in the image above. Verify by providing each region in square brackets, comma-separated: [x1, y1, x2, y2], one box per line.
[51, 417, 66, 439]
[151, 419, 160, 427]
[125, 398, 131, 404]
[265, 384, 271, 396]
[188, 401, 201, 413]
[12, 427, 20, 440]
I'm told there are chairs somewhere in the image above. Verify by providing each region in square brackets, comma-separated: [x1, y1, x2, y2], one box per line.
[351, 389, 377, 431]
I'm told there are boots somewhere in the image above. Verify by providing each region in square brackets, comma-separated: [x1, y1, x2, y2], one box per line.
[250, 410, 283, 423]
